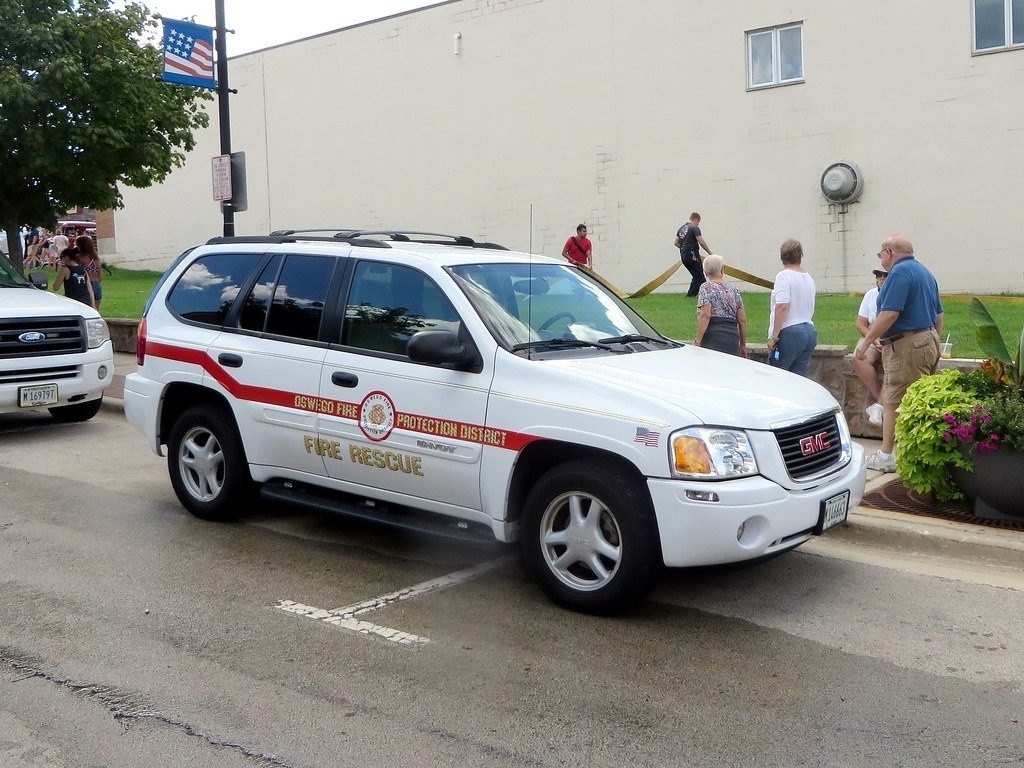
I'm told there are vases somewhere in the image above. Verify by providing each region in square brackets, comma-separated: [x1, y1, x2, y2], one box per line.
[949, 440, 1024, 515]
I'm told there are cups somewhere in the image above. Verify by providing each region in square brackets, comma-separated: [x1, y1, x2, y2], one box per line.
[940, 343, 953, 358]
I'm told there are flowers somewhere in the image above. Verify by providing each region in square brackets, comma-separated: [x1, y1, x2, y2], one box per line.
[890, 296, 1024, 504]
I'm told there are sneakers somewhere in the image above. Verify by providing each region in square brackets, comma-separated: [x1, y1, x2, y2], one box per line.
[866, 402, 883, 427]
[867, 450, 897, 473]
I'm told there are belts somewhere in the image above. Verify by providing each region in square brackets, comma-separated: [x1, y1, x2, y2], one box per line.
[879, 327, 935, 346]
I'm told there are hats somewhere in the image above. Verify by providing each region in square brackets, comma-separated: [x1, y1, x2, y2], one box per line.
[872, 266, 888, 274]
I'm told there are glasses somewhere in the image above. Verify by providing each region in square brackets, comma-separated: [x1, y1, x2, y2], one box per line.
[876, 272, 888, 278]
[877, 248, 892, 258]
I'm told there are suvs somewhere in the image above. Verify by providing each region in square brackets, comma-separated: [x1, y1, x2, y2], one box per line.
[123, 228, 868, 619]
[0, 252, 116, 424]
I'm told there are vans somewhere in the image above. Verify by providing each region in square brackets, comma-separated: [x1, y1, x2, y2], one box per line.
[56, 220, 97, 248]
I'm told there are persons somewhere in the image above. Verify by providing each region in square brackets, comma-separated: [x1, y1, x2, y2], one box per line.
[692, 254, 747, 359]
[17, 222, 103, 310]
[855, 233, 944, 473]
[562, 224, 593, 269]
[850, 261, 891, 426]
[767, 239, 818, 377]
[675, 213, 714, 297]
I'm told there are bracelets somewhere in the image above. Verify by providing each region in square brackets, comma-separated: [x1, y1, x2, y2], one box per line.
[772, 334, 780, 342]
[570, 259, 574, 262]
[695, 339, 701, 344]
